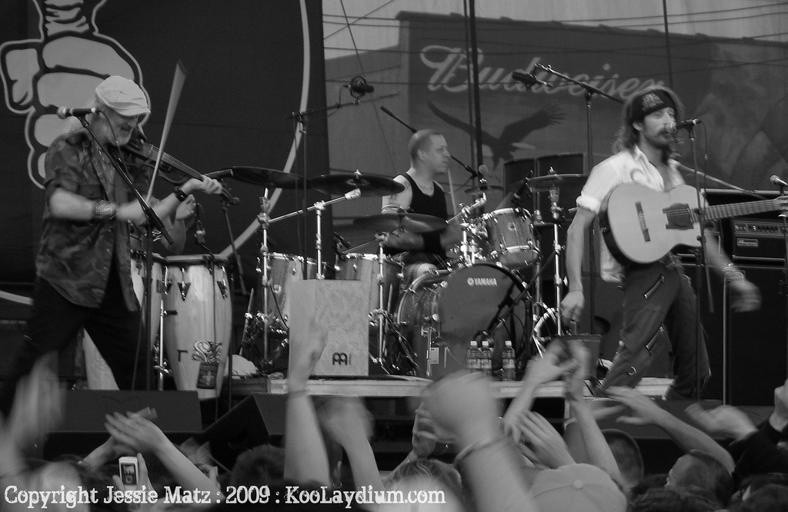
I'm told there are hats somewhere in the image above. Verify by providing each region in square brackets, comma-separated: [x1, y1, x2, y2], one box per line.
[93, 73, 152, 118]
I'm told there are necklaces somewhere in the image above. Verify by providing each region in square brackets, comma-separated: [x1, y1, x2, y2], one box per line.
[411, 175, 435, 194]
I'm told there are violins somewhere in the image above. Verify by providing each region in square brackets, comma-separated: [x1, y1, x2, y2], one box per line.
[122, 136, 241, 207]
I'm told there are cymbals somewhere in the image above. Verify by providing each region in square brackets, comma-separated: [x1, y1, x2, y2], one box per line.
[352, 207, 450, 234]
[309, 167, 406, 198]
[526, 173, 586, 186]
[223, 165, 306, 190]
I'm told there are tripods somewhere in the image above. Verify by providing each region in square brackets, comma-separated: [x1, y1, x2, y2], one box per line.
[238, 224, 290, 374]
[259, 276, 392, 378]
[368, 306, 427, 376]
[530, 300, 573, 354]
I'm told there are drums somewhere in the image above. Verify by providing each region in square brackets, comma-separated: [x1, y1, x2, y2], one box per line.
[82, 249, 168, 392]
[478, 205, 544, 272]
[254, 250, 327, 333]
[396, 260, 535, 385]
[334, 249, 403, 321]
[165, 252, 233, 403]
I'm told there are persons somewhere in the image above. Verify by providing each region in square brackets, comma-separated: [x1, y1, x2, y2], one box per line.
[556, 379, 788, 512]
[0, 356, 91, 512]
[382, 130, 451, 263]
[558, 87, 760, 403]
[94, 412, 281, 512]
[30, 75, 220, 389]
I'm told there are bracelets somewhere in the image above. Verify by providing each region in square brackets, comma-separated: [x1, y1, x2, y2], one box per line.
[174, 188, 187, 202]
[95, 200, 118, 222]
[720, 262, 745, 284]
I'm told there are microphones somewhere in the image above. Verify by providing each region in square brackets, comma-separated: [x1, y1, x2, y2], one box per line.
[511, 70, 553, 89]
[665, 117, 703, 137]
[54, 105, 97, 121]
[344, 81, 377, 94]
[478, 162, 489, 190]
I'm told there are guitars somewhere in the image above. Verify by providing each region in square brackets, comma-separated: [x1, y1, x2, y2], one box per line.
[597, 181, 784, 273]
